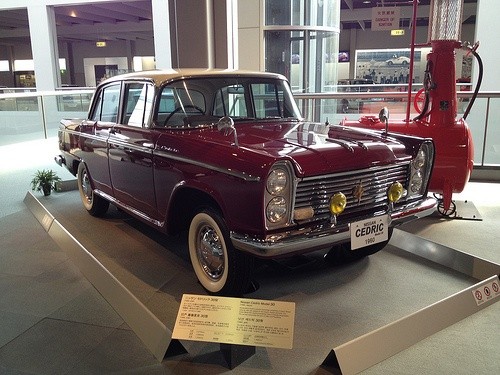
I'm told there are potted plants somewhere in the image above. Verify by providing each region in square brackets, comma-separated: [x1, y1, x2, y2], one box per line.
[31, 170, 62, 196]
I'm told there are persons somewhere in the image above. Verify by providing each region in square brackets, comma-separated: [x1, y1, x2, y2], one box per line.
[364, 66, 410, 85]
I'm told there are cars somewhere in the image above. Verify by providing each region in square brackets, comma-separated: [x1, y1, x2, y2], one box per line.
[55, 68, 438, 295]
[337, 78, 384, 111]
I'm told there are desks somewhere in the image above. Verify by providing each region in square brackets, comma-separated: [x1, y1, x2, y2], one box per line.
[338, 79, 374, 113]
